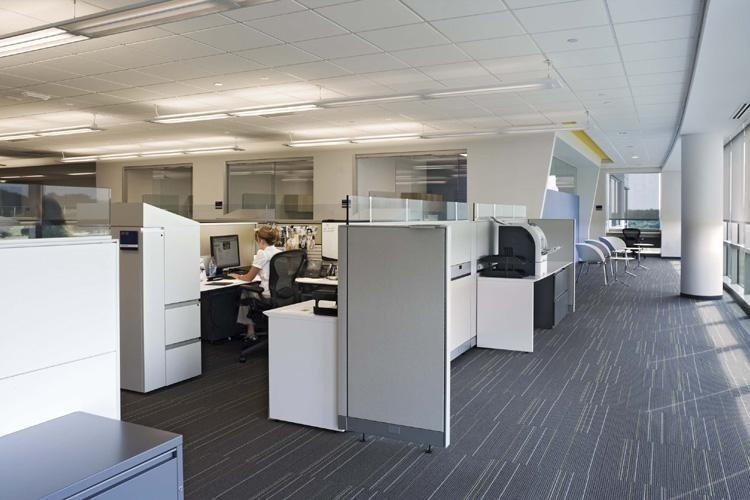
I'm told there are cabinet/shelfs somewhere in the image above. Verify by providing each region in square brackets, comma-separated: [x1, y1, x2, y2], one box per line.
[534, 267, 570, 329]
[0, 410, 184, 500]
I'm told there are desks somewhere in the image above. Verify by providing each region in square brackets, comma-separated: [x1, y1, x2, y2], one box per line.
[475, 261, 572, 352]
[633, 243, 654, 270]
[200, 266, 339, 293]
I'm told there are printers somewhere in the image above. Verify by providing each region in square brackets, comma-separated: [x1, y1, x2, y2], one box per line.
[498, 222, 561, 276]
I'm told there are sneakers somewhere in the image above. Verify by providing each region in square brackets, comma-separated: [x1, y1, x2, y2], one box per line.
[241, 335, 259, 346]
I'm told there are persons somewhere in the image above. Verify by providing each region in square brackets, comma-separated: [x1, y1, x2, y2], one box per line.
[225, 224, 286, 345]
[276, 226, 317, 251]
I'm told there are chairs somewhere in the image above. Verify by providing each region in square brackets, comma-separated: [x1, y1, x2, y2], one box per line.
[237, 249, 309, 364]
[574, 228, 644, 285]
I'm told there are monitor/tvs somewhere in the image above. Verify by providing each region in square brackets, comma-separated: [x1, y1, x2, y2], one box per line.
[210, 235, 240, 274]
